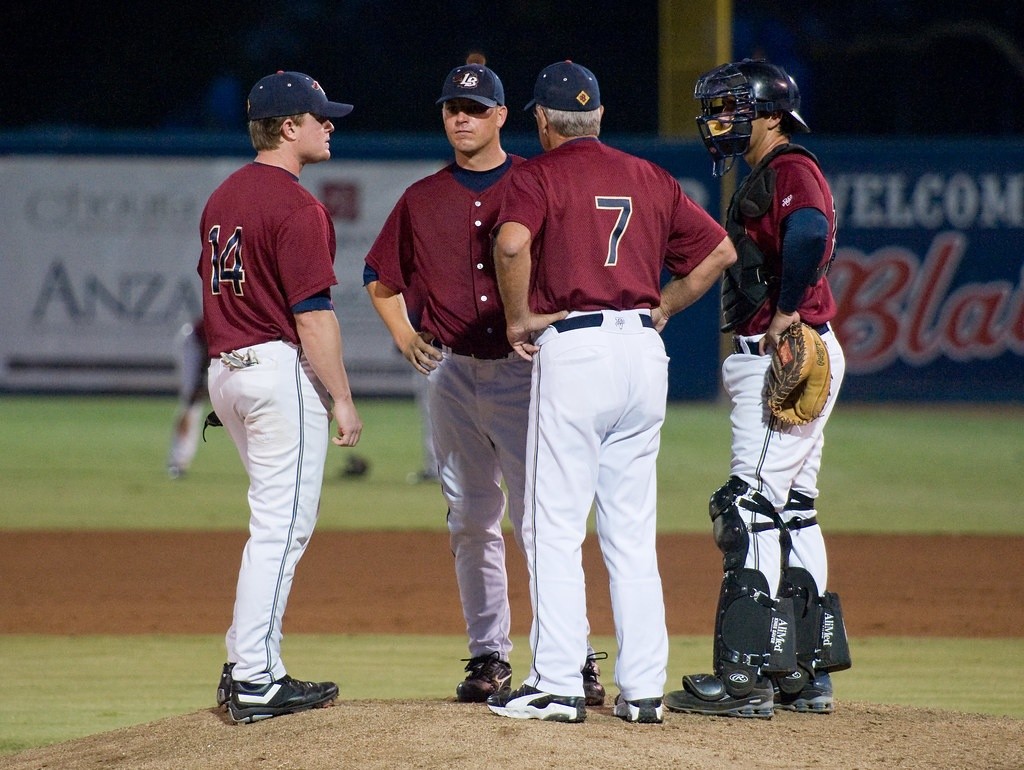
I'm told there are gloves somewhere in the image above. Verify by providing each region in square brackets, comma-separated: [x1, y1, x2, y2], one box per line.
[219, 348, 257, 371]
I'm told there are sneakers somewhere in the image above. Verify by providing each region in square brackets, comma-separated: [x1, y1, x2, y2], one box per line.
[229, 675, 339, 725]
[772, 670, 834, 714]
[457, 651, 512, 702]
[217, 663, 237, 707]
[664, 677, 774, 720]
[582, 652, 608, 705]
[614, 694, 664, 722]
[487, 683, 587, 723]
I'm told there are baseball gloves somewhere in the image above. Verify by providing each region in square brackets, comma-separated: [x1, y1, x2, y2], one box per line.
[206, 409, 223, 427]
[761, 323, 833, 427]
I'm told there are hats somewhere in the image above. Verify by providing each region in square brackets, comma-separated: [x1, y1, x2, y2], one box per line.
[436, 63, 504, 107]
[247, 70, 354, 120]
[524, 61, 601, 111]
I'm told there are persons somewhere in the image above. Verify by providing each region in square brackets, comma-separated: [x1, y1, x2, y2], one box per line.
[198, 71, 362, 724]
[406, 362, 442, 482]
[486, 59, 738, 726]
[168, 319, 211, 477]
[660, 59, 844, 720]
[362, 52, 603, 708]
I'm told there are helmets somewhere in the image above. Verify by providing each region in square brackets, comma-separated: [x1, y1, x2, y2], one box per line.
[717, 59, 811, 133]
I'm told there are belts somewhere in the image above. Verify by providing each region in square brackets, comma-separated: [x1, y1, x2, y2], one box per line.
[433, 338, 508, 359]
[733, 322, 829, 355]
[551, 314, 656, 333]
